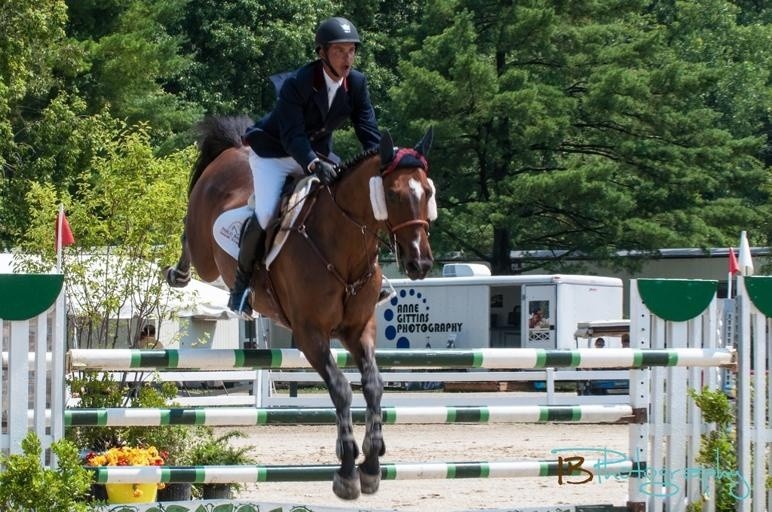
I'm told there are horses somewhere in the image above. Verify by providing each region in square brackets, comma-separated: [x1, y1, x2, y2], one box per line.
[159, 109, 435, 501]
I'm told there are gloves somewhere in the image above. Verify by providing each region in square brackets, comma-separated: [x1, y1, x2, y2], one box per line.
[310, 159, 337, 185]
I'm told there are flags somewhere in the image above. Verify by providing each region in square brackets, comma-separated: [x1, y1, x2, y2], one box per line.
[729, 250, 740, 275]
[739, 231, 756, 277]
[52, 210, 77, 254]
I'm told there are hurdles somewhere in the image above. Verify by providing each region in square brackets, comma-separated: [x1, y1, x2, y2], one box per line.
[0, 269, 740, 511]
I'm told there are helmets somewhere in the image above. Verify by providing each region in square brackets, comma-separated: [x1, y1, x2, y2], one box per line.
[315, 17, 359, 50]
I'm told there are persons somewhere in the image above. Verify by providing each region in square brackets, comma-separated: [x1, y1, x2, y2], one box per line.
[136, 324, 164, 349]
[225, 16, 384, 321]
[534, 306, 549, 328]
[528, 311, 539, 330]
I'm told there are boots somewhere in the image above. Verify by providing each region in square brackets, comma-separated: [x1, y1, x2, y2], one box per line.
[228, 210, 268, 314]
